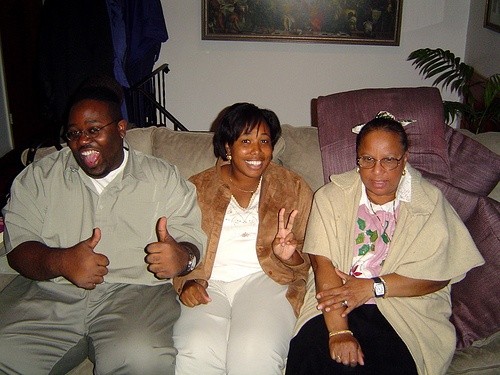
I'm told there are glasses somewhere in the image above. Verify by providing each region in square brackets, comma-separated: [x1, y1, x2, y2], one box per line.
[64, 120, 117, 142]
[356, 149, 406, 169]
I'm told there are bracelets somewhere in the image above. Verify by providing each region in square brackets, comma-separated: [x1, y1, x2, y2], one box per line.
[380, 277, 387, 299]
[329, 330, 353, 337]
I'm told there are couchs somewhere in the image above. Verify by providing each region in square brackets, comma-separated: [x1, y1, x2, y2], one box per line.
[0, 127, 500, 375]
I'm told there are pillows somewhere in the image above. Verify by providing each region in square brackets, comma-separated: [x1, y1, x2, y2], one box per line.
[448, 196, 500, 347]
[421, 172, 500, 224]
[440, 124, 500, 196]
[316, 87, 452, 184]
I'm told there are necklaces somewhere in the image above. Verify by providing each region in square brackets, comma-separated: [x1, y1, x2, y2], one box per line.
[370, 199, 396, 243]
[226, 172, 258, 192]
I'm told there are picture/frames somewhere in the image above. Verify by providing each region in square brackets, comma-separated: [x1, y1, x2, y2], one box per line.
[483, 0, 500, 33]
[200, 0, 404, 46]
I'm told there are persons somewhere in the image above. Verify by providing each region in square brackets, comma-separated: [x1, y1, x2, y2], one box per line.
[0, 75, 208, 375]
[173, 103, 315, 375]
[286, 117, 486, 375]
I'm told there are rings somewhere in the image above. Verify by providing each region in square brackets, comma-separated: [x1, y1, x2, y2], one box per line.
[336, 356, 341, 360]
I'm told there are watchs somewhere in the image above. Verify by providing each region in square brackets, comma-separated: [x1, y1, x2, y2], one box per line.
[371, 276, 384, 298]
[179, 245, 197, 276]
